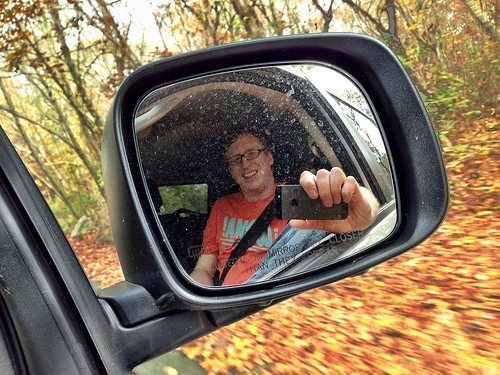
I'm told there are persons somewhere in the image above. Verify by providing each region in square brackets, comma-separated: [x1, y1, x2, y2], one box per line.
[185, 125, 381, 286]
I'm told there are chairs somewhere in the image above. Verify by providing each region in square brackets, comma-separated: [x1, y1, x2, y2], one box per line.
[145, 178, 193, 278]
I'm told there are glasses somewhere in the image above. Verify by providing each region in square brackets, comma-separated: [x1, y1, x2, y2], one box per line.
[228, 147, 269, 166]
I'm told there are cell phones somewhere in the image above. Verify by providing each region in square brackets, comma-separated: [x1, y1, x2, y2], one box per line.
[275, 186, 349, 221]
